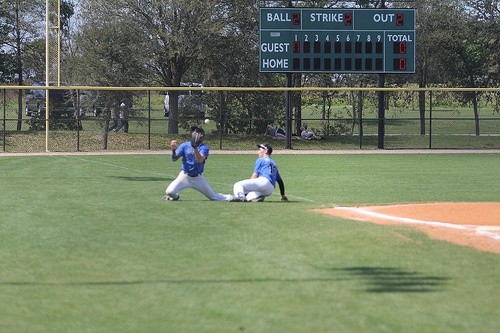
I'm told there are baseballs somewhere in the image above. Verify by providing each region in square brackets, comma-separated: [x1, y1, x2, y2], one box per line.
[205, 119, 209, 123]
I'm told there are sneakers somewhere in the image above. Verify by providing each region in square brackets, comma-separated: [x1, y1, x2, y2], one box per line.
[162, 194, 179, 201]
[249, 195, 264, 203]
[231, 198, 245, 202]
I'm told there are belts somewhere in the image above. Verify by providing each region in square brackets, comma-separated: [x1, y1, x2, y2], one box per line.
[184, 172, 201, 177]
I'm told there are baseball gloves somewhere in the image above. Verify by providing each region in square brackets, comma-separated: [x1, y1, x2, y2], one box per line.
[191, 132, 204, 148]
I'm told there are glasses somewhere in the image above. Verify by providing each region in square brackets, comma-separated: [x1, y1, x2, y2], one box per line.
[191, 129, 200, 133]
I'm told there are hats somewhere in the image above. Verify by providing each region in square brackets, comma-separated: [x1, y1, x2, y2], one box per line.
[257, 143, 272, 154]
[190, 125, 204, 131]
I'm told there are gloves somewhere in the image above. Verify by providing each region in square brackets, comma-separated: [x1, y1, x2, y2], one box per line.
[191, 138, 198, 148]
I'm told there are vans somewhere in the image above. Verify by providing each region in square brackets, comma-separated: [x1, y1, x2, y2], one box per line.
[25, 81, 75, 116]
[163, 82, 206, 118]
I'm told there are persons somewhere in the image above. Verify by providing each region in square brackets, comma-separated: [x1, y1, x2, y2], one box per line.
[113, 98, 128, 133]
[165, 128, 233, 201]
[104, 99, 119, 131]
[266, 122, 317, 139]
[230, 143, 288, 202]
